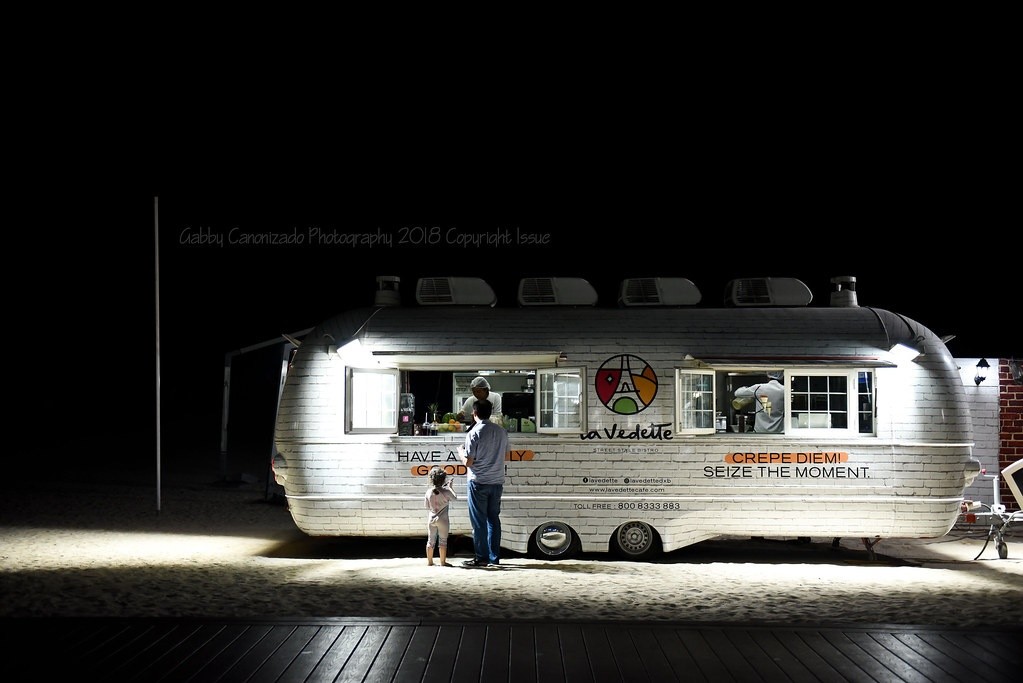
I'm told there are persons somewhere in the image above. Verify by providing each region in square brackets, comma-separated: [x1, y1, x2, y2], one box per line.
[457, 377, 501, 420]
[457, 399, 510, 569]
[424, 468, 457, 567]
[734, 370, 784, 433]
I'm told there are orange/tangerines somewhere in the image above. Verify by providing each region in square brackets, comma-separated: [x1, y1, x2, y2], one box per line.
[440, 419, 460, 433]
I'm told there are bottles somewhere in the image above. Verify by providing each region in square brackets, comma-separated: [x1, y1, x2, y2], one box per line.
[423, 413, 430, 436]
[430, 413, 438, 436]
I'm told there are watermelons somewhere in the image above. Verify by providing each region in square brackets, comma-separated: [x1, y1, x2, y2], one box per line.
[442, 413, 456, 423]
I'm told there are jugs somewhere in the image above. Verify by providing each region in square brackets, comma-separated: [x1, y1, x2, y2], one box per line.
[731, 397, 754, 410]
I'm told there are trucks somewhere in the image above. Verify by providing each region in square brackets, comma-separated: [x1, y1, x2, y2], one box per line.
[270, 274, 985, 563]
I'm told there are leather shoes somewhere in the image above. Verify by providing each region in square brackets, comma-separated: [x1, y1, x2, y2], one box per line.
[463, 558, 499, 567]
